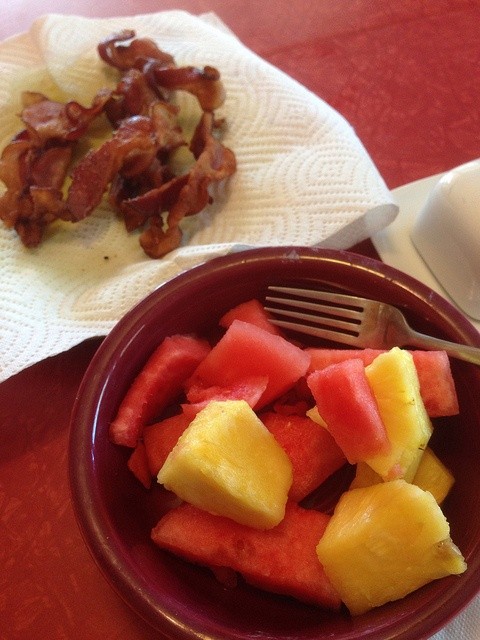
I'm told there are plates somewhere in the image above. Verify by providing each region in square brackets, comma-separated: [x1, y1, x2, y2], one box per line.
[371, 156, 479, 336]
[67, 246, 479, 640]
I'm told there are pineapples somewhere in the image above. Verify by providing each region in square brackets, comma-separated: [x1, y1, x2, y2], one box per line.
[349, 446, 456, 506]
[305, 345, 435, 484]
[157, 400, 295, 531]
[316, 479, 468, 615]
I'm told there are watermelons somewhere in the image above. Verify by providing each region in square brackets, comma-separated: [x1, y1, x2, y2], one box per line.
[307, 358, 392, 465]
[296, 348, 460, 418]
[252, 410, 347, 504]
[128, 443, 152, 489]
[182, 319, 311, 413]
[218, 299, 287, 340]
[109, 334, 212, 448]
[142, 375, 270, 477]
[151, 501, 342, 610]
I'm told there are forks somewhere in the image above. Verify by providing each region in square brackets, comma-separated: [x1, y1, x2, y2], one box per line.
[262, 286, 480, 361]
